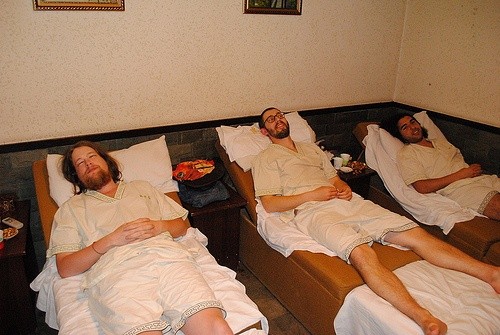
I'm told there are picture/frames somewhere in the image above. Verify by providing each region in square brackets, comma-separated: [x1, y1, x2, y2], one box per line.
[33, 0, 125, 11]
[242, 0, 303, 16]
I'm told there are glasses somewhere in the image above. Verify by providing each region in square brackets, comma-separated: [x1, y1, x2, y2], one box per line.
[262, 112, 285, 125]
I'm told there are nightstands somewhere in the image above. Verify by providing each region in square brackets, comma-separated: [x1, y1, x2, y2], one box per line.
[0, 199, 40, 310]
[179, 192, 249, 272]
[329, 159, 378, 202]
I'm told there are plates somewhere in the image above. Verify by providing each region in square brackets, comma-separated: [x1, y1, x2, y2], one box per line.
[2, 228, 19, 240]
[340, 166, 353, 174]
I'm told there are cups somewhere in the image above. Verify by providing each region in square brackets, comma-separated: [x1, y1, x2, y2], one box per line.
[333, 157, 343, 170]
[341, 153, 350, 167]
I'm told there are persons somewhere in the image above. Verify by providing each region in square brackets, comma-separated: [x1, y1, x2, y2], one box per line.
[376, 113, 500, 222]
[45, 140, 235, 335]
[251, 106, 500, 335]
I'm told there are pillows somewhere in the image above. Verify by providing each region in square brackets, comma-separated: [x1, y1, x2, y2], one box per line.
[46, 134, 179, 204]
[217, 110, 321, 171]
[367, 110, 448, 153]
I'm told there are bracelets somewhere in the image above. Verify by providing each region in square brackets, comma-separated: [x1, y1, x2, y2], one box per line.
[93, 242, 107, 256]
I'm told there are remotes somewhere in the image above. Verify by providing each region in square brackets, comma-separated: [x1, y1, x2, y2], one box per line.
[2, 217, 23, 229]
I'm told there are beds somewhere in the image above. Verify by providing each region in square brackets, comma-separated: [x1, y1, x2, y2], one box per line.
[215, 140, 500, 335]
[350, 121, 500, 268]
[32, 157, 263, 335]
